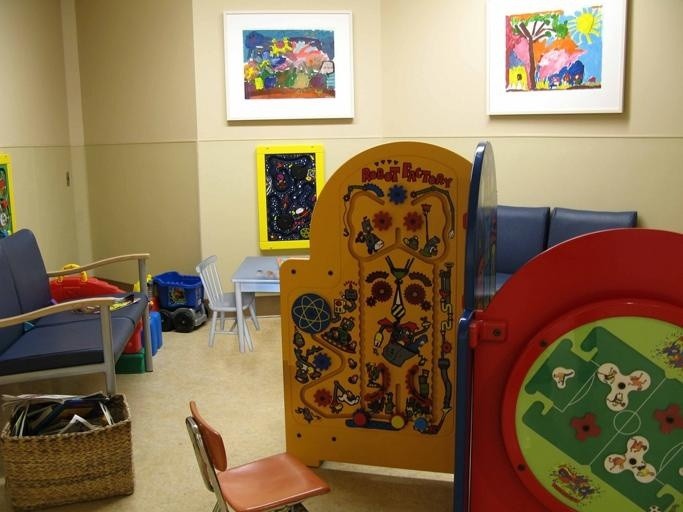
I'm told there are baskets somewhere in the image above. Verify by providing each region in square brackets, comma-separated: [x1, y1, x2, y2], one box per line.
[2, 394, 134, 511]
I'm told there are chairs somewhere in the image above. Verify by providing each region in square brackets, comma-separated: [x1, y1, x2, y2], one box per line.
[196, 254, 260, 352]
[184, 401, 330, 511]
[489, 204, 637, 294]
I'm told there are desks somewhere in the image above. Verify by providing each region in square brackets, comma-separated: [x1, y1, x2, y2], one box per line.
[231, 253, 309, 352]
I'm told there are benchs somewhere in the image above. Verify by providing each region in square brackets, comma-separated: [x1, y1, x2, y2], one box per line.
[1, 227, 153, 393]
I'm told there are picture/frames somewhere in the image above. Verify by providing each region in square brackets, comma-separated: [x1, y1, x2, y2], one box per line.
[0, 153, 16, 238]
[222, 9, 353, 121]
[484, 1, 628, 115]
[256, 143, 325, 252]
